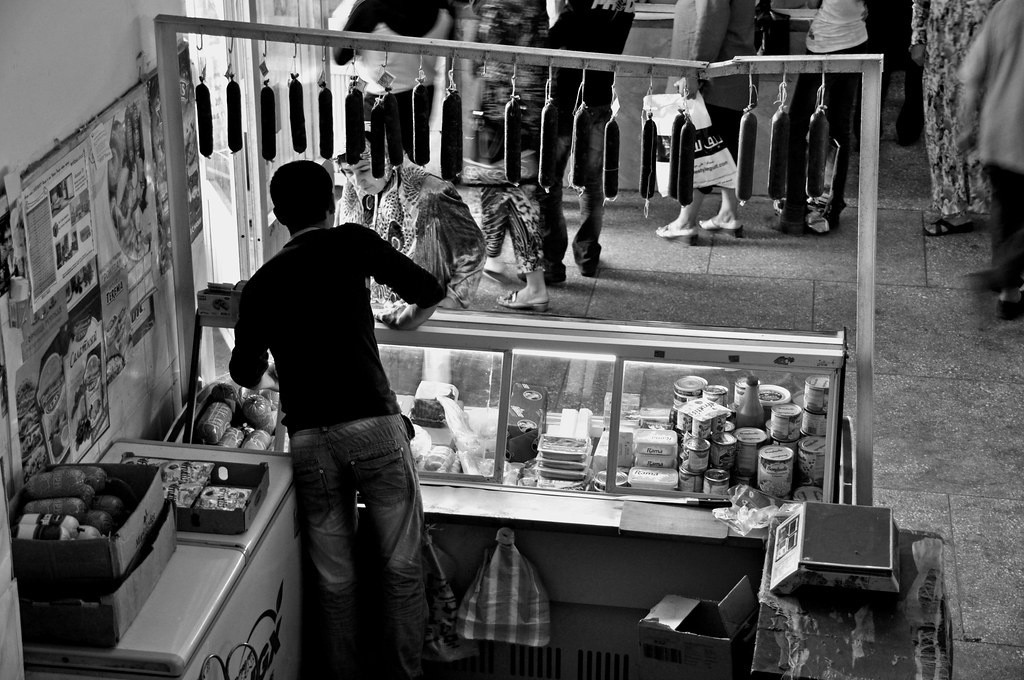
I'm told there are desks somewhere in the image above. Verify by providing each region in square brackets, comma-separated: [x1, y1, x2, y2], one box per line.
[751, 514, 955, 680]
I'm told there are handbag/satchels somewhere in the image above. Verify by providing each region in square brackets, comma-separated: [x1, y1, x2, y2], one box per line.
[641, 90, 737, 198]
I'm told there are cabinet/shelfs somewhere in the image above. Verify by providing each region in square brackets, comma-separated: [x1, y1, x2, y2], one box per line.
[185, 288, 851, 679]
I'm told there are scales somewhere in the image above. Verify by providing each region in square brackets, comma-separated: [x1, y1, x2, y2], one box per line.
[769, 501, 901, 598]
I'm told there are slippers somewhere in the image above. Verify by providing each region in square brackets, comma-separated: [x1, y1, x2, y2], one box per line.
[921, 217, 975, 237]
[483, 269, 512, 285]
[495, 290, 550, 312]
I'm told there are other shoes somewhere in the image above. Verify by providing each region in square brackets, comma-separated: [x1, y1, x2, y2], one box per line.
[772, 196, 826, 214]
[996, 289, 1024, 321]
[516, 264, 567, 283]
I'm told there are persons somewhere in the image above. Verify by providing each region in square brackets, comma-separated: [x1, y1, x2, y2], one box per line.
[951, 0, 1023, 322]
[227, 158, 450, 680]
[770, 0, 872, 213]
[906, 0, 1004, 238]
[330, 119, 489, 312]
[512, 0, 637, 283]
[654, 0, 760, 246]
[455, 0, 551, 312]
[325, 0, 453, 150]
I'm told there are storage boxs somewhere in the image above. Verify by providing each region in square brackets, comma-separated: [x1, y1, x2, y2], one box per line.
[117, 452, 270, 536]
[7, 462, 178, 651]
[636, 575, 758, 680]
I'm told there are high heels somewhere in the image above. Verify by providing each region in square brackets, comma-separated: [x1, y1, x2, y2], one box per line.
[698, 217, 744, 239]
[655, 223, 699, 246]
